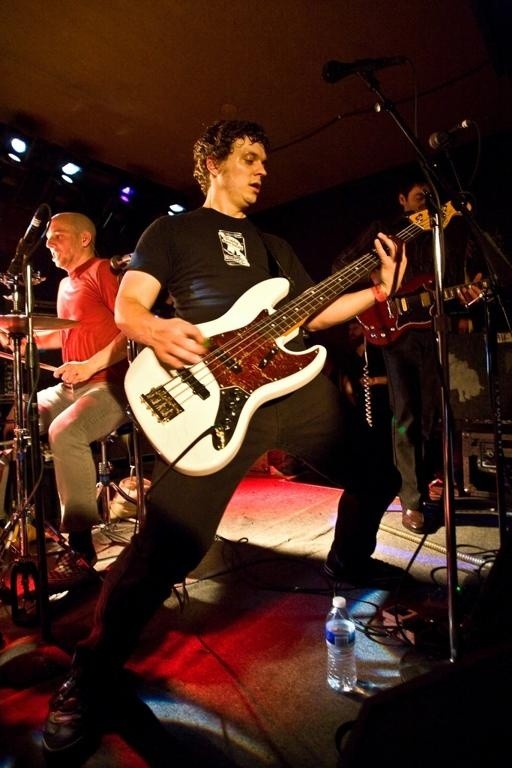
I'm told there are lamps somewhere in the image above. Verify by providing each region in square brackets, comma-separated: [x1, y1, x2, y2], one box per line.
[162, 195, 188, 216]
[8, 133, 34, 167]
[116, 171, 140, 204]
[59, 158, 84, 185]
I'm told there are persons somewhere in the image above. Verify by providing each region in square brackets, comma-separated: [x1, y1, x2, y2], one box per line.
[0, 212, 129, 586]
[334, 173, 483, 532]
[344, 321, 389, 409]
[42, 118, 410, 755]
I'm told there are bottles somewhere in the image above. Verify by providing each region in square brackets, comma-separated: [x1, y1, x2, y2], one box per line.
[326, 597, 356, 693]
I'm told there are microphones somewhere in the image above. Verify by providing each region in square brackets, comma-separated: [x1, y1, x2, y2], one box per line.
[320, 52, 396, 81]
[5, 206, 51, 274]
[426, 115, 476, 147]
[108, 253, 138, 269]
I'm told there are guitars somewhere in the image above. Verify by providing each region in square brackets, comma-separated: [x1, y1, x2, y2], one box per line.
[124, 200, 472, 478]
[355, 276, 495, 344]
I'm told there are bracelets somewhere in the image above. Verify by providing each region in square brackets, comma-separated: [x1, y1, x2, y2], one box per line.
[371, 283, 388, 304]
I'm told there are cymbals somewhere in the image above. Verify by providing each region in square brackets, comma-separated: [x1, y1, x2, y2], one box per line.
[0, 315, 82, 329]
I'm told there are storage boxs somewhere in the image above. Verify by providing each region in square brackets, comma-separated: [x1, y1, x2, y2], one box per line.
[461, 419, 512, 500]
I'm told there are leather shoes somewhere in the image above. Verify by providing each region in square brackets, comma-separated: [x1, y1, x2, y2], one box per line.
[401, 506, 428, 535]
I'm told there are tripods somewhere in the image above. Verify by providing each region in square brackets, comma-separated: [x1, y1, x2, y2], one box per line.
[0, 340, 96, 588]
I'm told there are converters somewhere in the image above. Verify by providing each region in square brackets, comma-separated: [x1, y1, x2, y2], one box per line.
[382, 613, 434, 645]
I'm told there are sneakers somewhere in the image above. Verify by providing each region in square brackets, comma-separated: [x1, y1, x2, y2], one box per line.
[37, 666, 107, 758]
[41, 547, 99, 585]
[323, 551, 417, 590]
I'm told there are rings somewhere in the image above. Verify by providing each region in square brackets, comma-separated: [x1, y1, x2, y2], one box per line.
[75, 373, 78, 375]
